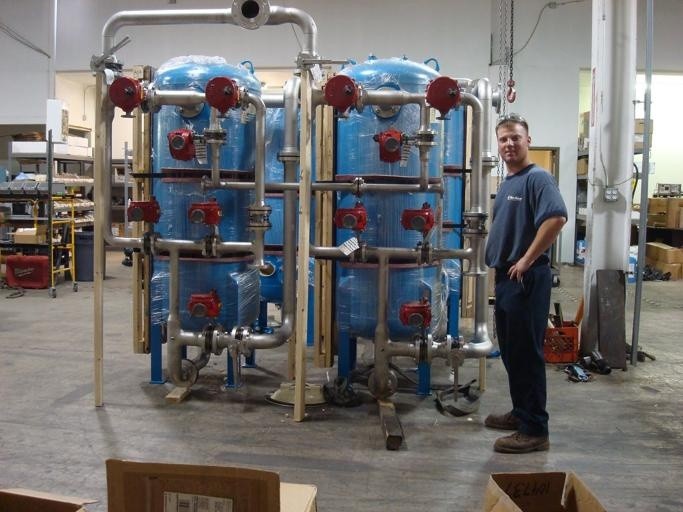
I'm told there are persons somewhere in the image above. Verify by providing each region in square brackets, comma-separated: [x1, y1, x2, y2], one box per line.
[484, 114, 568, 454]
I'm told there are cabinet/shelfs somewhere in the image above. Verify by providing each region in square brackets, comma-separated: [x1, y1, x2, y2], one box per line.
[1, 176, 66, 267]
[111, 158, 137, 240]
[13, 151, 94, 255]
[576, 116, 658, 226]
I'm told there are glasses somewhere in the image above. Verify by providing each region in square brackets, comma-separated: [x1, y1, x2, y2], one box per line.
[496, 111, 525, 125]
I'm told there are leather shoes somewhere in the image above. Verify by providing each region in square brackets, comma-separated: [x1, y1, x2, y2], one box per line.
[484, 411, 518, 430]
[493, 432, 549, 454]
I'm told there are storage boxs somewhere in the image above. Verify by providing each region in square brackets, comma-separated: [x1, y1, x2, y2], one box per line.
[648, 197, 683, 230]
[580, 111, 590, 122]
[5, 227, 47, 245]
[658, 246, 683, 264]
[634, 133, 652, 148]
[482, 469, 606, 512]
[634, 119, 653, 134]
[656, 261, 683, 281]
[105, 458, 319, 512]
[5, 254, 48, 288]
[527, 149, 553, 173]
[578, 120, 589, 138]
[577, 160, 587, 174]
[645, 257, 657, 268]
[0, 487, 99, 512]
[646, 241, 670, 259]
